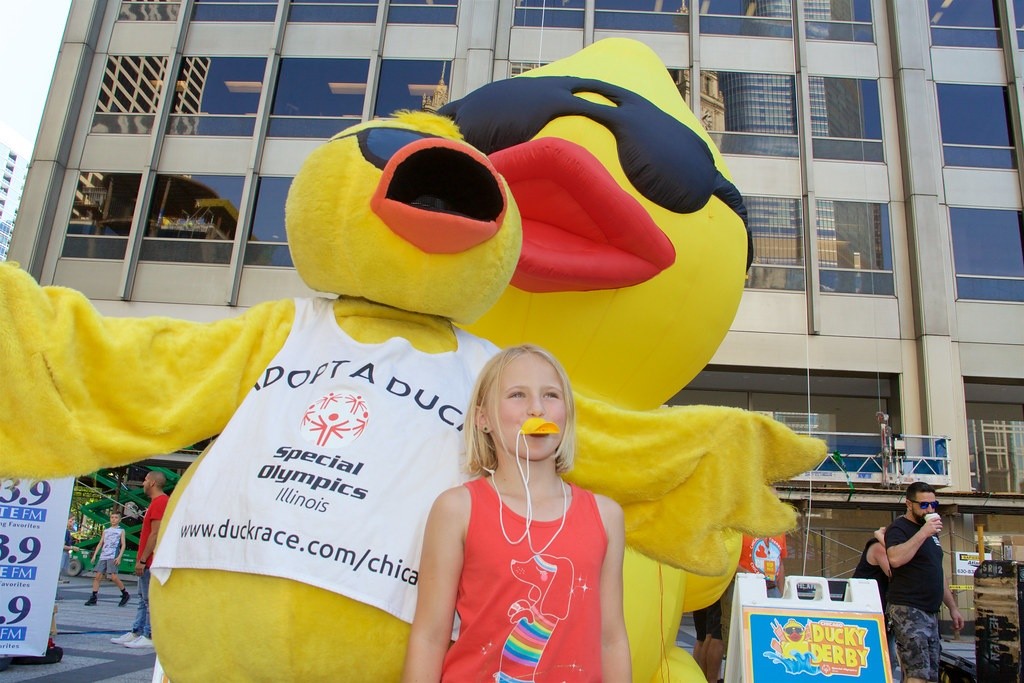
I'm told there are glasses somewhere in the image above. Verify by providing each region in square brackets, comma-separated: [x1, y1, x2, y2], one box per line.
[910, 500, 939, 509]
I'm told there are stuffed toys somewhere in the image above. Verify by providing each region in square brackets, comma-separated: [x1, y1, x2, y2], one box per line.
[435, 39, 753, 683]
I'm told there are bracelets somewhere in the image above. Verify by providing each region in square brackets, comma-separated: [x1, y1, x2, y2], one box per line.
[71, 546, 72, 550]
[140, 560, 147, 564]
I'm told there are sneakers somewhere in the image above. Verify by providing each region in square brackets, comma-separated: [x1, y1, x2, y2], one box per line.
[118, 592, 130, 607]
[124, 635, 154, 648]
[85, 595, 97, 606]
[111, 632, 138, 643]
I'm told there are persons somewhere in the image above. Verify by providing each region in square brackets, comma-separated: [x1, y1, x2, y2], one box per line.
[55, 511, 79, 600]
[693, 600, 724, 683]
[111, 472, 169, 648]
[403, 346, 632, 683]
[0, 110, 826, 682]
[86, 511, 131, 607]
[852, 528, 899, 675]
[734, 485, 788, 598]
[884, 482, 964, 683]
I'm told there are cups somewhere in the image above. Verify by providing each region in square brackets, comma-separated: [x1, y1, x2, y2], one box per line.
[924, 513, 942, 532]
